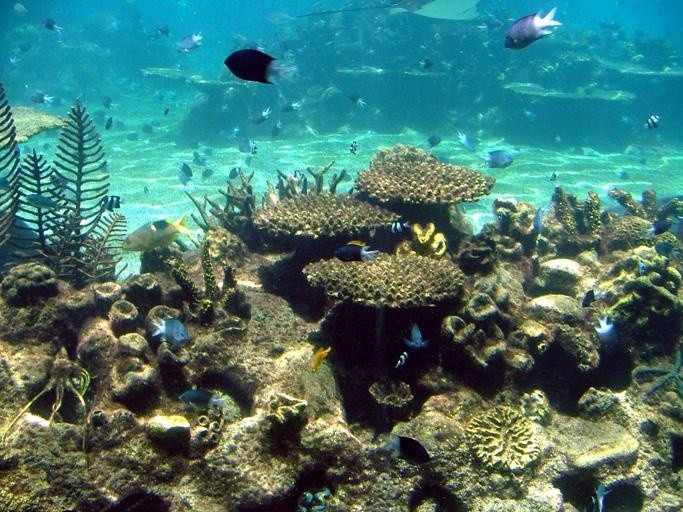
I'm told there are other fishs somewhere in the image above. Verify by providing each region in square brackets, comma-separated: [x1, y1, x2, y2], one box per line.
[0, 0, 683, 309]
